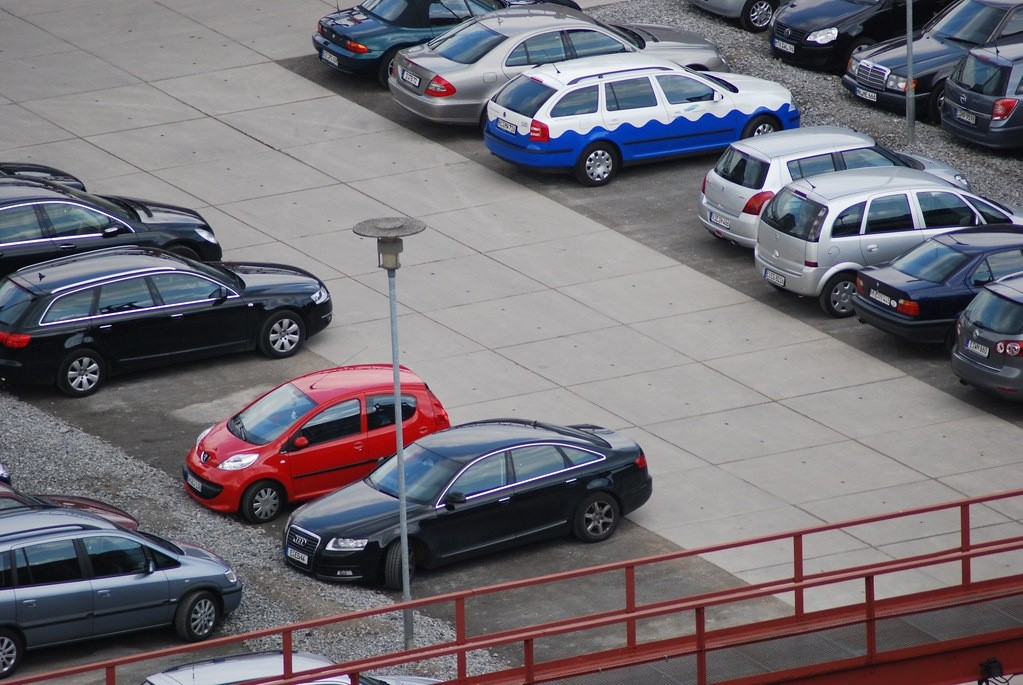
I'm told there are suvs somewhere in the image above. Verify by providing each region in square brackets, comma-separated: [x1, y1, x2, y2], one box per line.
[0, 244, 334, 399]
[951, 272, 1023, 404]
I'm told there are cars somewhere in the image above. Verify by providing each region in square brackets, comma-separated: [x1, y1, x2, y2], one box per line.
[689, 0, 792, 34]
[769, 0, 953, 78]
[841, 0, 1023, 123]
[851, 225, 1023, 356]
[0, 163, 85, 191]
[281, 418, 653, 591]
[312, 0, 583, 90]
[483, 54, 801, 187]
[752, 166, 1023, 319]
[698, 125, 973, 251]
[0, 468, 244, 679]
[938, 34, 1023, 152]
[387, 4, 731, 135]
[0, 175, 223, 283]
[180, 365, 451, 525]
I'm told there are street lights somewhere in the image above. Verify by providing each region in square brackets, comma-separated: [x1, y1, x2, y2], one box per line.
[352, 219, 428, 649]
